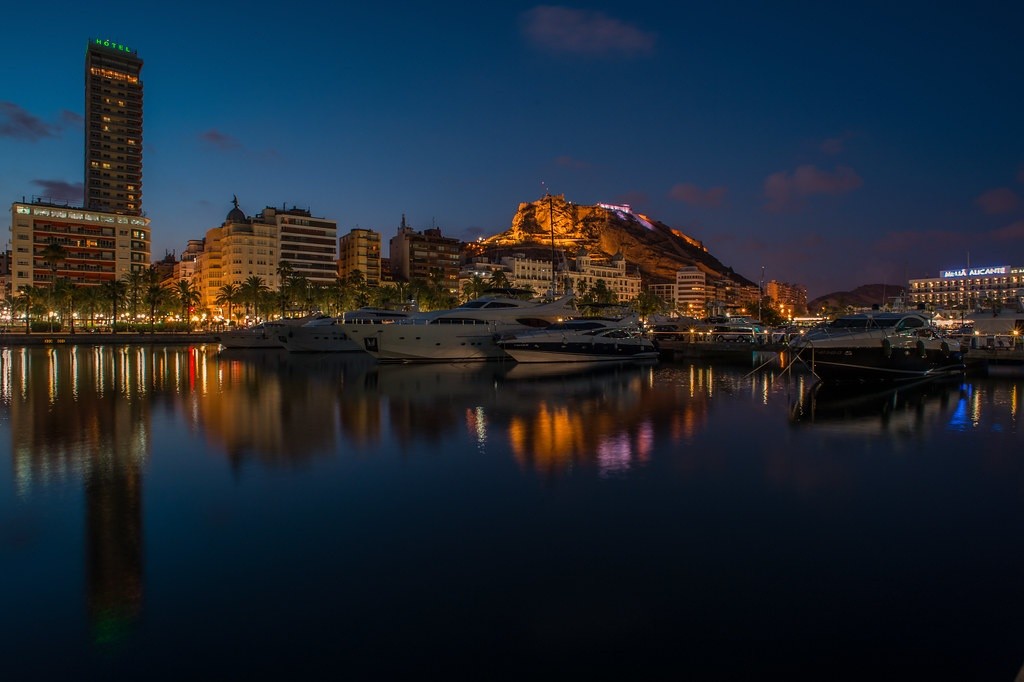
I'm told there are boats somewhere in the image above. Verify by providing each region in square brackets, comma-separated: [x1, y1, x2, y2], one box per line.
[210, 312, 323, 349]
[496, 312, 660, 364]
[264, 306, 422, 353]
[787, 312, 969, 383]
[788, 374, 963, 436]
[333, 288, 583, 362]
[210, 346, 655, 416]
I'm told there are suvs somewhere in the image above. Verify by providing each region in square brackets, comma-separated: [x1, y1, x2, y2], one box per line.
[717, 328, 753, 342]
